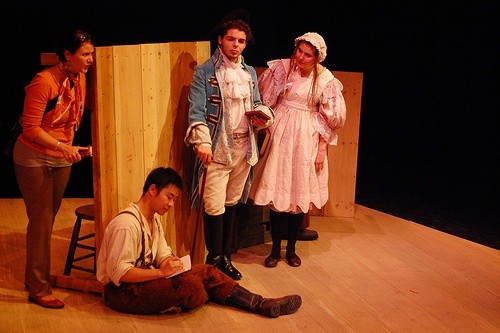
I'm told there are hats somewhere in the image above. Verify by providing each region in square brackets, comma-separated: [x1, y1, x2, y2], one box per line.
[295, 31, 327, 62]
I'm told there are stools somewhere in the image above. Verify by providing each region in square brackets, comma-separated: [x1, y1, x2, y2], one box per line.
[64, 206, 97, 276]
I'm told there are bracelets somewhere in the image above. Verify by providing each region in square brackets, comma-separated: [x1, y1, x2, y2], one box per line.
[199, 144, 211, 148]
[54, 141, 60, 150]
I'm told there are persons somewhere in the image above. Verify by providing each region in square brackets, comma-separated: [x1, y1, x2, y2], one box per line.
[247, 32, 347, 267]
[184, 19, 276, 280]
[95, 167, 303, 318]
[12, 30, 95, 308]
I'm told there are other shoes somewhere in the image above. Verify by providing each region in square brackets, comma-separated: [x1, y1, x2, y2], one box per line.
[28, 295, 65, 309]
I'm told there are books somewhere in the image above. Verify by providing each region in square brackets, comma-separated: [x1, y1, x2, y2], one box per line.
[165, 255, 192, 279]
[257, 127, 271, 156]
[78, 145, 92, 158]
[244, 109, 271, 120]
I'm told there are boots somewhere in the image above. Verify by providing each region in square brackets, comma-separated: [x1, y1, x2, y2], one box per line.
[227, 283, 302, 319]
[264, 209, 302, 268]
[204, 203, 243, 281]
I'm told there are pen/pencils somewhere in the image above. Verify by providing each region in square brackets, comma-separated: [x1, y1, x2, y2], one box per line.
[171, 251, 176, 259]
